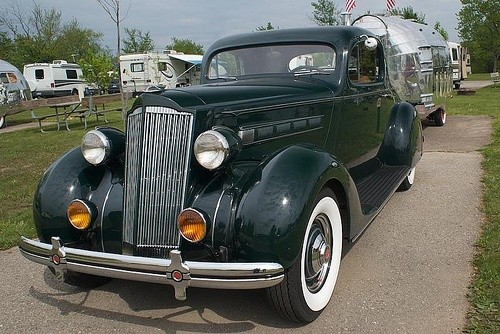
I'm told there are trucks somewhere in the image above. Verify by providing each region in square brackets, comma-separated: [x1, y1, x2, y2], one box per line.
[22, 57, 98, 98]
[117, 46, 229, 98]
[0, 58, 33, 130]
[448, 41, 475, 92]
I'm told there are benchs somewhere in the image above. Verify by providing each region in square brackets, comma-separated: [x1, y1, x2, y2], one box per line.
[30, 109, 90, 134]
[72, 108, 124, 125]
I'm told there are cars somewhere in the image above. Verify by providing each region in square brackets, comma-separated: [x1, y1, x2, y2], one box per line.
[15, 22, 425, 326]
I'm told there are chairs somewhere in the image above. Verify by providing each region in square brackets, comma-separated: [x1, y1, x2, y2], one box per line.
[264, 52, 281, 71]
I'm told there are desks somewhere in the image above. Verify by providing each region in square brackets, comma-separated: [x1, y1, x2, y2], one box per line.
[92, 93, 126, 123]
[39, 102, 88, 134]
